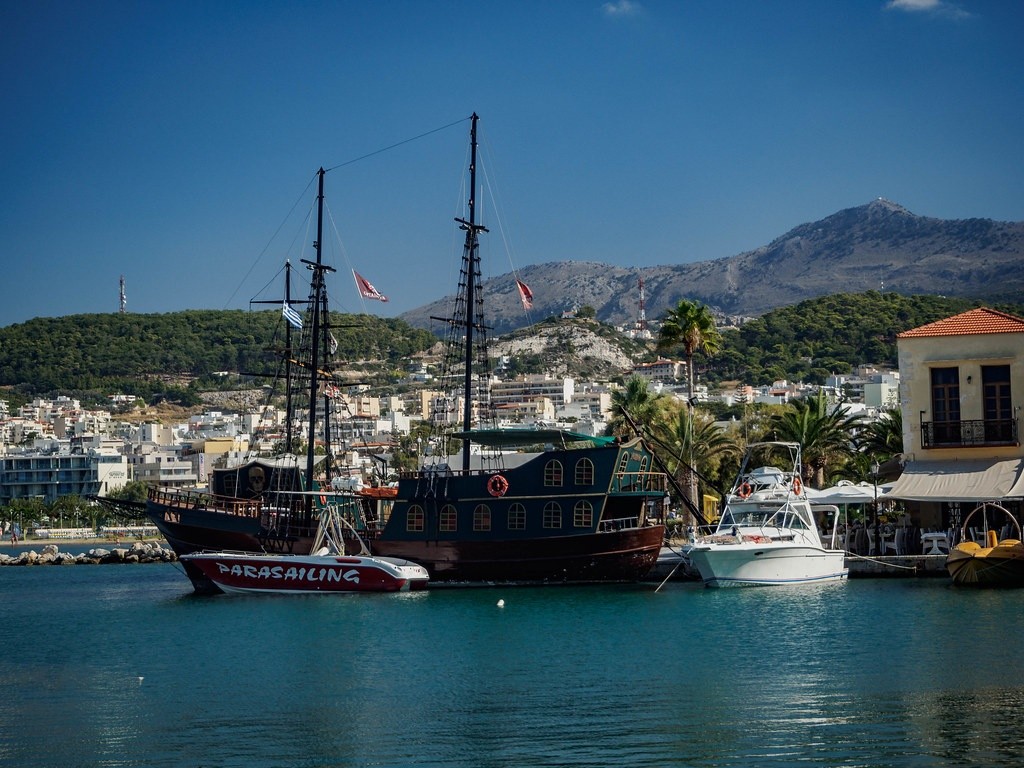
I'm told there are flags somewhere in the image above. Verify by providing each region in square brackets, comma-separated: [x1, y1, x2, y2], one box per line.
[515, 278, 534, 312]
[352, 267, 389, 302]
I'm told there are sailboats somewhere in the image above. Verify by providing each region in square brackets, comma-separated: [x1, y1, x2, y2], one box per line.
[81, 112, 672, 602]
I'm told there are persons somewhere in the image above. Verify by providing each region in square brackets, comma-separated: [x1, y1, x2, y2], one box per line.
[10, 533, 18, 547]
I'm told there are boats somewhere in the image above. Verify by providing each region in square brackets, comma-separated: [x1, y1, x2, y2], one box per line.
[670, 440, 850, 590]
[945, 501, 1023, 585]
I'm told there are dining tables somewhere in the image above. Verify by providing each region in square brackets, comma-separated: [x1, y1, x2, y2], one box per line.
[816, 527, 1022, 556]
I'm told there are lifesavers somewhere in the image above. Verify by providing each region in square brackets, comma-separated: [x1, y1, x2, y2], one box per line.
[320, 488, 327, 505]
[487, 474, 507, 497]
[738, 483, 752, 498]
[793, 478, 801, 493]
